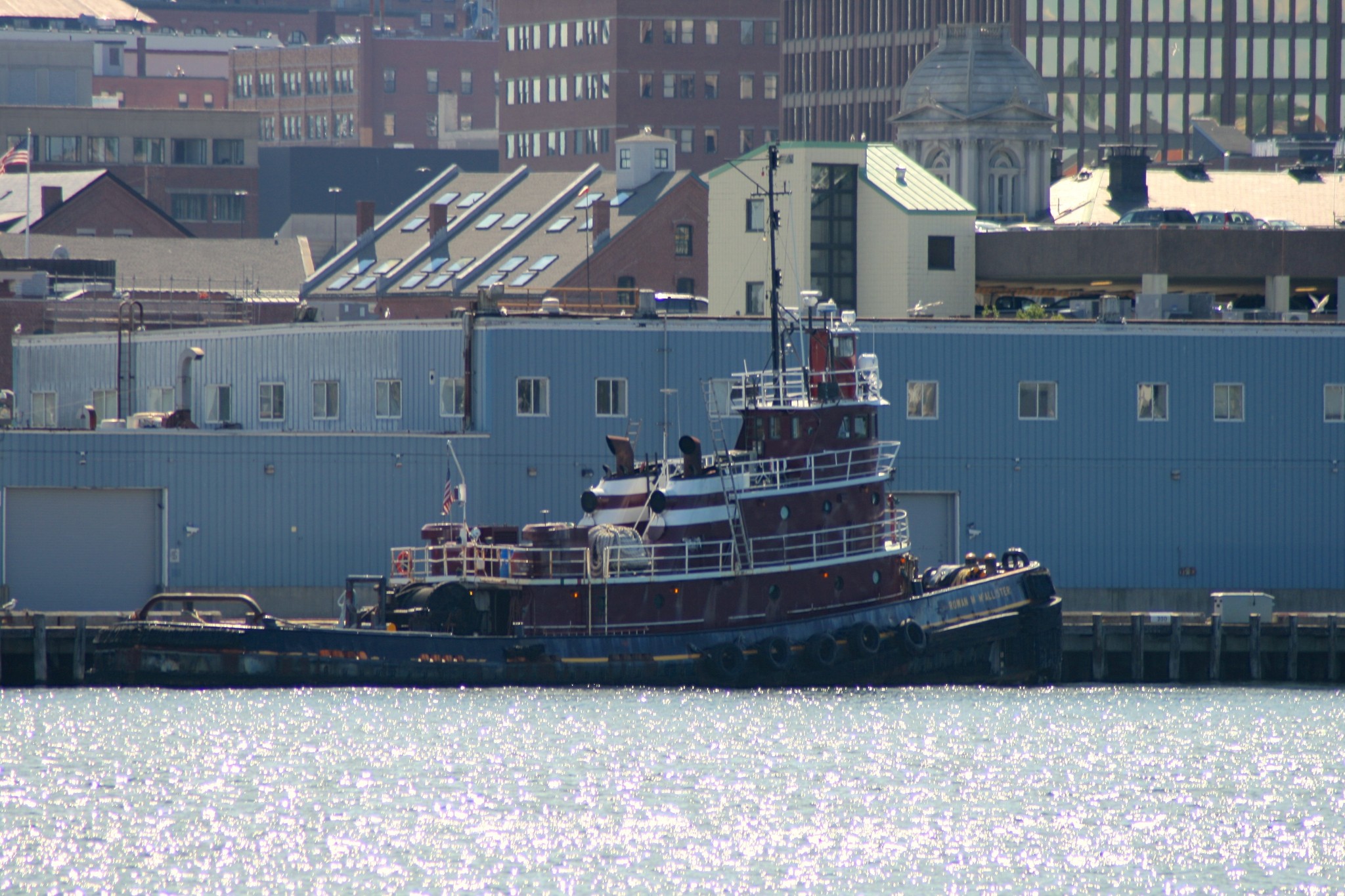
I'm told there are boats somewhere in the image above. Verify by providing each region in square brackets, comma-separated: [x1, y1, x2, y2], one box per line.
[85, 145, 1067, 689]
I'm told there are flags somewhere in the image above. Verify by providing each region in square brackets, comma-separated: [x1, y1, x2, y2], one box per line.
[0, 135, 28, 175]
[441, 458, 453, 515]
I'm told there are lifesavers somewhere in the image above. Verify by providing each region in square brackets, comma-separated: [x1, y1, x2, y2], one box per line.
[896, 618, 927, 654]
[848, 621, 881, 659]
[805, 632, 838, 670]
[397, 551, 413, 577]
[758, 635, 792, 671]
[706, 641, 744, 682]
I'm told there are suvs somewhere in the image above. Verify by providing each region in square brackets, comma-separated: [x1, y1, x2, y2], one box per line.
[1115, 206, 1193, 227]
[994, 294, 1036, 320]
[654, 293, 708, 315]
[1192, 211, 1257, 228]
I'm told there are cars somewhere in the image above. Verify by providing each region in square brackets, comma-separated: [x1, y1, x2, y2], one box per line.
[1265, 219, 1303, 231]
[1042, 294, 1102, 318]
[1005, 224, 1047, 232]
[975, 220, 1005, 233]
[1212, 293, 1245, 316]
